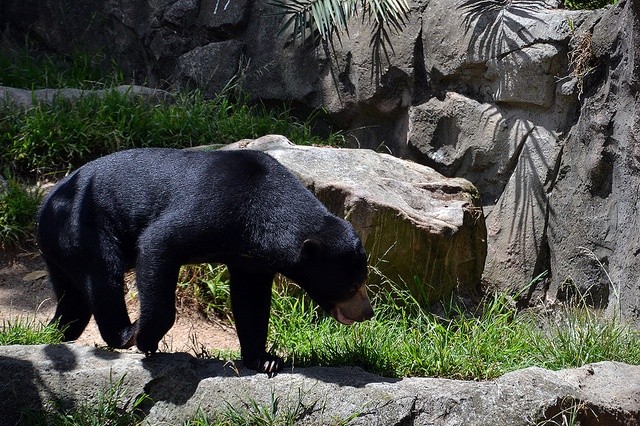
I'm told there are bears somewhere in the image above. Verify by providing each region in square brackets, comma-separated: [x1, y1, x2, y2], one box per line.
[35, 147, 374, 374]
